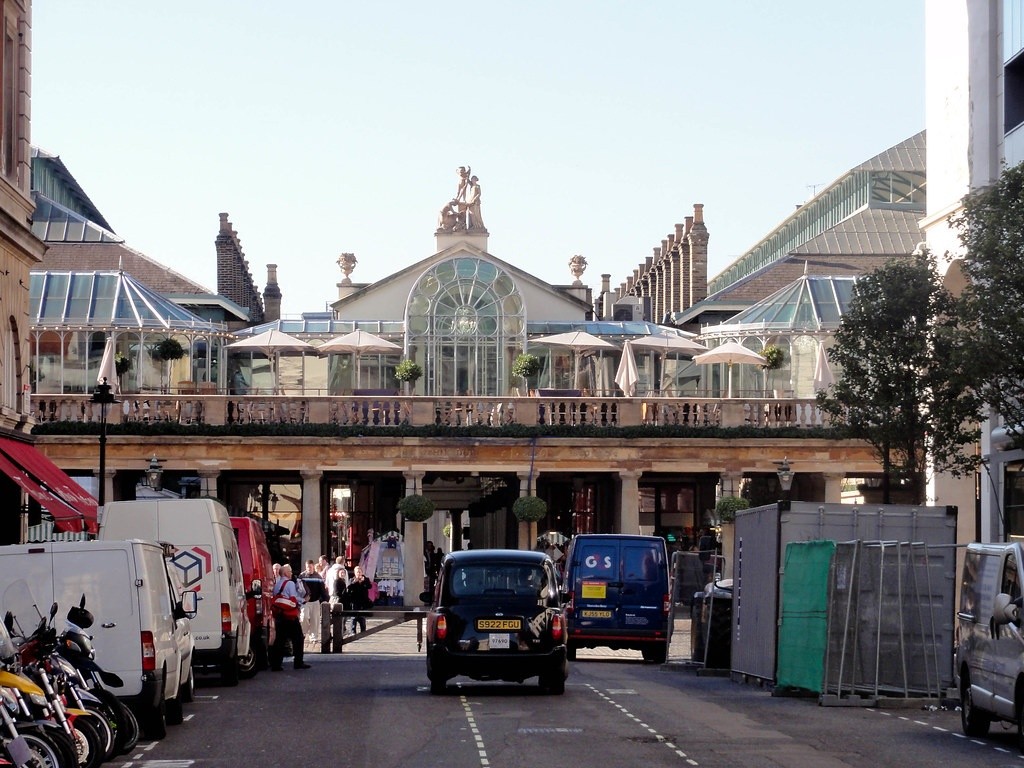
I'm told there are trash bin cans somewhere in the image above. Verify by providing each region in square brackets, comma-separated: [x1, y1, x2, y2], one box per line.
[690, 591, 732, 668]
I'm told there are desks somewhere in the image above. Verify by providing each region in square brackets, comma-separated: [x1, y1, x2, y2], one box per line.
[539, 387, 582, 402]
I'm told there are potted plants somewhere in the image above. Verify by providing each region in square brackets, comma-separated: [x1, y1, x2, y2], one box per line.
[111, 351, 131, 374]
[514, 496, 547, 522]
[758, 346, 783, 368]
[154, 339, 185, 360]
[513, 354, 541, 378]
[399, 496, 437, 523]
[396, 360, 422, 380]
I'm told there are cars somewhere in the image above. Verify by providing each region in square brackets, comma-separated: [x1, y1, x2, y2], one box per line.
[419, 549, 571, 697]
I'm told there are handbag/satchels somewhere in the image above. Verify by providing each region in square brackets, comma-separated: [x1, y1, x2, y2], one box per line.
[270, 580, 300, 621]
[362, 578, 380, 603]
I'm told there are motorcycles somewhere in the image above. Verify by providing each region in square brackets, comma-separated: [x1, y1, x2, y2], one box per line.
[0, 593, 140, 767]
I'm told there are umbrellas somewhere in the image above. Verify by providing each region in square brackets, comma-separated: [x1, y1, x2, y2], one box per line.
[527, 329, 614, 389]
[813, 340, 836, 392]
[97, 335, 117, 394]
[314, 328, 402, 389]
[222, 329, 315, 394]
[691, 341, 767, 398]
[615, 339, 639, 398]
[622, 329, 712, 397]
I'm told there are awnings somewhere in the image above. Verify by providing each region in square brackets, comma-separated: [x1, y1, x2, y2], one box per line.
[0, 437, 99, 534]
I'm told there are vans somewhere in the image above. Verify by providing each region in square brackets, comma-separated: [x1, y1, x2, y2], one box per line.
[0, 498, 277, 701]
[953, 541, 1024, 753]
[556, 533, 676, 664]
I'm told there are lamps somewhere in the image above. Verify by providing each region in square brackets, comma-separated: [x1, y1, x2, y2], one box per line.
[137, 457, 167, 487]
[775, 458, 795, 492]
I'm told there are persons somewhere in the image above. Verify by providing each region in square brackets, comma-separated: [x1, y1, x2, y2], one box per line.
[272, 564, 312, 672]
[298, 559, 325, 647]
[466, 175, 484, 227]
[436, 547, 445, 579]
[315, 555, 372, 636]
[452, 166, 469, 220]
[424, 540, 435, 592]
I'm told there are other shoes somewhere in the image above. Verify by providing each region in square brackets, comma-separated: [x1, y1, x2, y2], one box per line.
[293, 664, 311, 670]
[271, 667, 284, 672]
